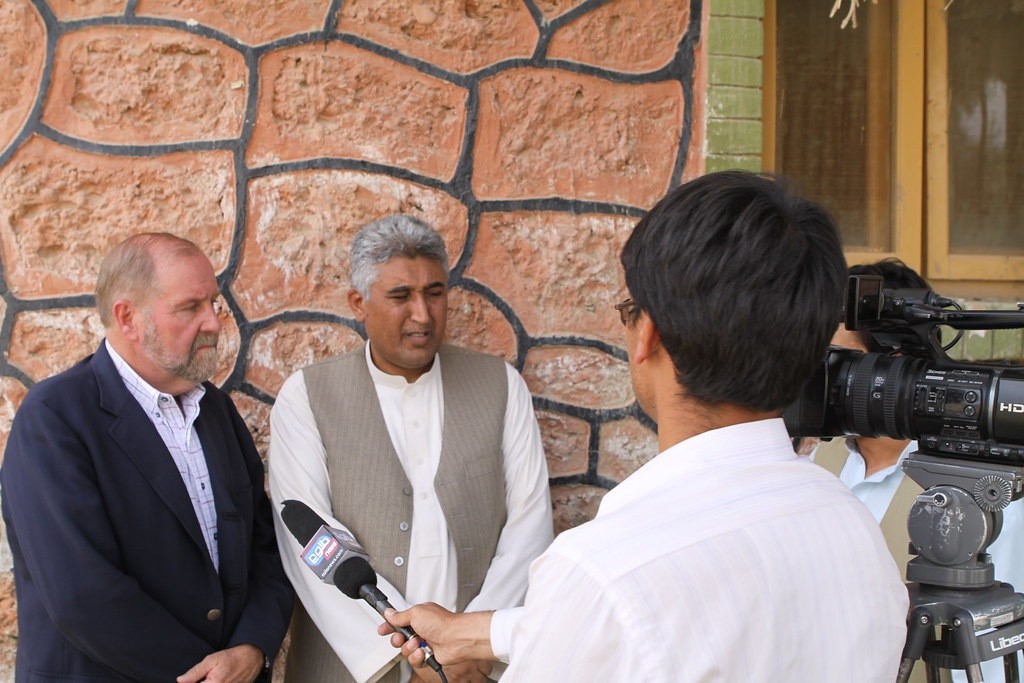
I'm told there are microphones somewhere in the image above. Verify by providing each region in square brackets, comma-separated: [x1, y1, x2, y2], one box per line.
[281, 499, 442, 672]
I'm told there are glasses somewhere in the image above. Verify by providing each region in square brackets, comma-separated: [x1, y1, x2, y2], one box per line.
[614, 298, 638, 327]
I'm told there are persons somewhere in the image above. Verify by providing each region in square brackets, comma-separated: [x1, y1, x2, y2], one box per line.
[378, 167, 909, 683]
[804, 260, 952, 683]
[269, 214, 556, 682]
[0, 232, 294, 683]
